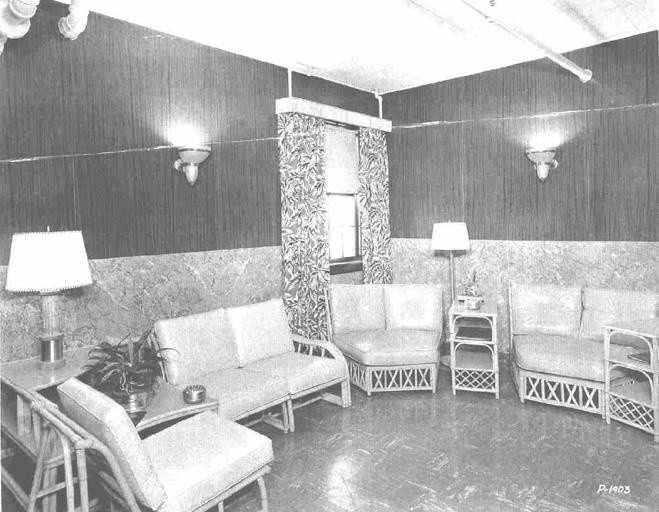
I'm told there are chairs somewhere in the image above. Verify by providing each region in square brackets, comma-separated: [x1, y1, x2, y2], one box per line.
[220, 370, 224, 375]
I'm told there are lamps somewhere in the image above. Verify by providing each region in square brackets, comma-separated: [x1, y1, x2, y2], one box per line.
[4, 229, 94, 372]
[524, 148, 560, 185]
[431, 222, 470, 303]
[174, 145, 212, 188]
